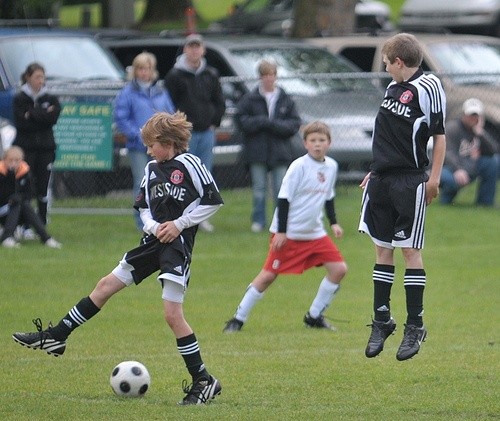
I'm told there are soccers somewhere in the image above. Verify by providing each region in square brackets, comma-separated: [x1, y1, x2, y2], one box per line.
[110, 361, 151, 397]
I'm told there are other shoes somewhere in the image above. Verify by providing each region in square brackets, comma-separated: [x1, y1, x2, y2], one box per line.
[251, 223, 262, 233]
[2, 237, 19, 248]
[303, 310, 339, 334]
[220, 318, 244, 333]
[201, 220, 214, 232]
[47, 237, 61, 248]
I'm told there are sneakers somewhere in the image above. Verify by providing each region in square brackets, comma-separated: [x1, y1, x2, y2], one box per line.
[364, 315, 397, 359]
[11, 318, 66, 357]
[176, 375, 222, 406]
[396, 322, 428, 361]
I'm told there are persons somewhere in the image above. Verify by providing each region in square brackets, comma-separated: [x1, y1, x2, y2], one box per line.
[359, 33, 446, 361]
[0, 62, 62, 250]
[438, 98, 500, 206]
[164, 34, 227, 233]
[222, 121, 349, 333]
[114, 51, 175, 238]
[13, 111, 222, 403]
[232, 61, 302, 232]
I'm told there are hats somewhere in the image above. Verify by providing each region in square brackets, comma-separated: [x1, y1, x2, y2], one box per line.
[185, 33, 202, 46]
[463, 98, 483, 116]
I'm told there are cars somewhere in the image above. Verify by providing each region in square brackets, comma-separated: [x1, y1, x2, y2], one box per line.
[92, 27, 389, 191]
[0, 17, 132, 201]
[298, 25, 500, 181]
